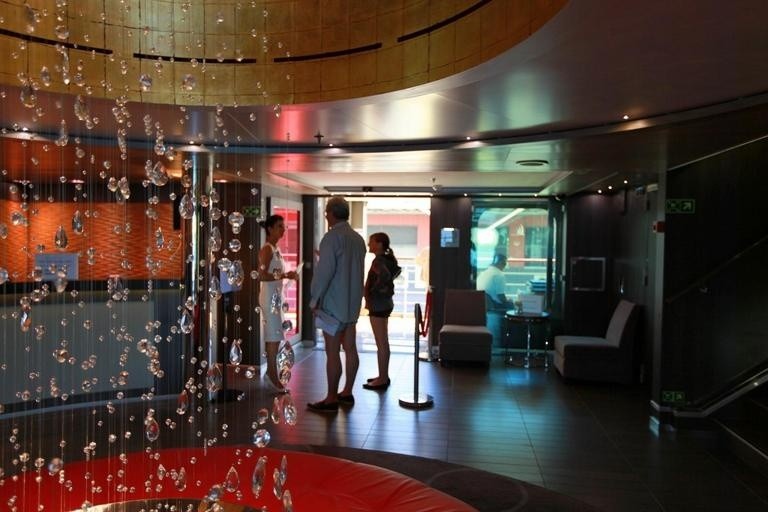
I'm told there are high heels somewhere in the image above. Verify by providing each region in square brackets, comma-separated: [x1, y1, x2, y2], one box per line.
[263, 373, 290, 394]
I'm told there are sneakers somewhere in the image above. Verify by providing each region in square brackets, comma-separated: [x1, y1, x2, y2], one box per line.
[306, 400, 339, 413]
[367, 377, 390, 385]
[338, 392, 354, 406]
[363, 382, 388, 391]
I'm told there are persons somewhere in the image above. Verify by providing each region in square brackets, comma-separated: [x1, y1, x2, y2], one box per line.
[476, 254, 514, 310]
[362, 232, 401, 391]
[306, 197, 367, 414]
[257, 213, 299, 394]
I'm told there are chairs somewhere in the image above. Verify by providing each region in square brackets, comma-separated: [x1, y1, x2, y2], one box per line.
[437, 287, 493, 369]
[555, 299, 647, 385]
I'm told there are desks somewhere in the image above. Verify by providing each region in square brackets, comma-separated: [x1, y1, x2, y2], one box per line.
[505, 309, 553, 369]
